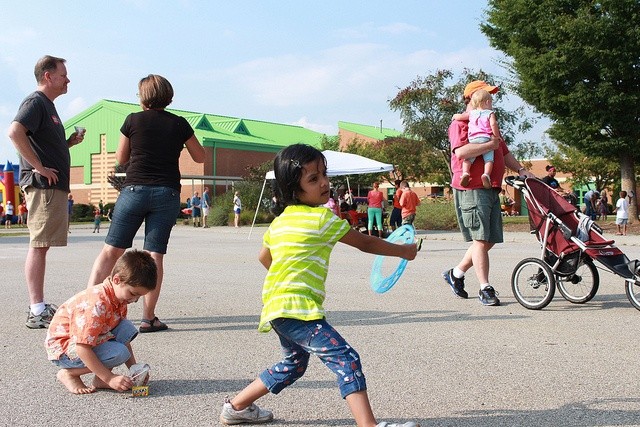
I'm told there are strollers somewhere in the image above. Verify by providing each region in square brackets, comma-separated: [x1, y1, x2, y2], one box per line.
[504, 175, 640, 313]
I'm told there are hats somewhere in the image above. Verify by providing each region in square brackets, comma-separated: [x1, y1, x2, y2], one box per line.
[464, 80, 498, 98]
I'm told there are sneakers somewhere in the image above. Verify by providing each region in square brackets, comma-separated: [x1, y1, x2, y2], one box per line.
[441, 268, 468, 299]
[460, 173, 470, 188]
[26, 304, 57, 329]
[479, 285, 499, 306]
[219, 395, 273, 425]
[481, 174, 492, 189]
[378, 421, 420, 427]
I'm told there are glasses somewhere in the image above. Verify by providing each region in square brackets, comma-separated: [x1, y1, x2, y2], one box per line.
[136, 92, 141, 98]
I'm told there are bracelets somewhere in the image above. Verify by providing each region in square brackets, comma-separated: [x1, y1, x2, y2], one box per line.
[517, 167, 527, 172]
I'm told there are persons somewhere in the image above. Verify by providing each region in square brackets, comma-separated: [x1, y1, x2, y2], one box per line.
[499, 190, 518, 217]
[542, 165, 564, 192]
[68, 191, 72, 233]
[451, 89, 501, 189]
[7, 54, 87, 328]
[584, 189, 628, 236]
[187, 187, 211, 228]
[0, 201, 27, 230]
[87, 73, 206, 333]
[439, 79, 536, 306]
[93, 198, 112, 233]
[268, 191, 283, 216]
[233, 189, 243, 228]
[44, 248, 158, 394]
[366, 179, 424, 251]
[219, 143, 418, 425]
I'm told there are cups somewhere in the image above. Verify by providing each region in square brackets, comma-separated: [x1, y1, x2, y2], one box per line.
[74, 126, 86, 141]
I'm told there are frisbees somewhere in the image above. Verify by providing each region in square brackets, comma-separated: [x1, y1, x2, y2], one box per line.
[370, 225, 414, 292]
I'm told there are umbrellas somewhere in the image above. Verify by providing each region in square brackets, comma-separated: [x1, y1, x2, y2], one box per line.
[266, 149, 394, 179]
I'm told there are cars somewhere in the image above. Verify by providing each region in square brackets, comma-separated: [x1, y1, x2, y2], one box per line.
[339, 197, 368, 226]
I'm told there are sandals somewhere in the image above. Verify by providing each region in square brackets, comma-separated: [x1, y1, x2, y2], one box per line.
[139, 317, 168, 333]
